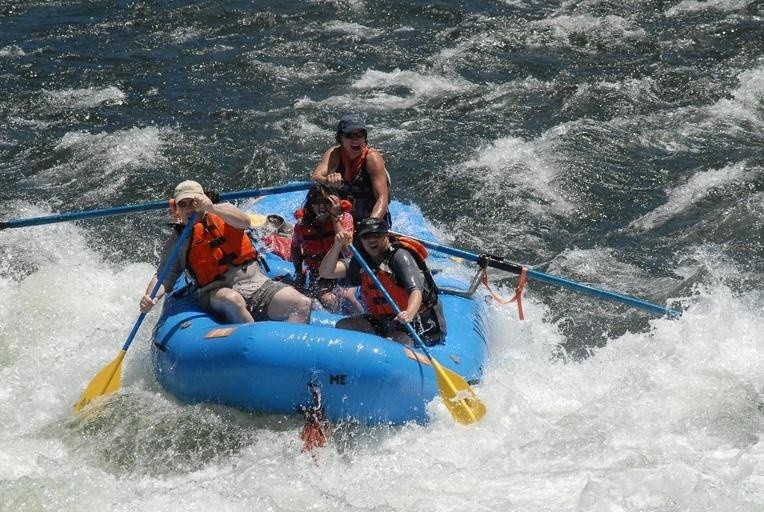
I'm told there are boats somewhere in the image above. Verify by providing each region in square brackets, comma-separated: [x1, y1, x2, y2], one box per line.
[151, 182, 490, 421]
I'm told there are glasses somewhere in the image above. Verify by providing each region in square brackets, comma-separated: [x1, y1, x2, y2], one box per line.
[178, 200, 193, 207]
[309, 198, 331, 204]
[345, 132, 363, 138]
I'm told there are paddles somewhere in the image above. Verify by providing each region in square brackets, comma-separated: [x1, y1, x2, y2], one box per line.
[74, 205, 200, 415]
[343, 239, 486, 427]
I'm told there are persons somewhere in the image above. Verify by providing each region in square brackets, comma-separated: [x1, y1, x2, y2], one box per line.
[139, 180, 311, 323]
[314, 113, 392, 229]
[319, 217, 448, 347]
[292, 182, 365, 314]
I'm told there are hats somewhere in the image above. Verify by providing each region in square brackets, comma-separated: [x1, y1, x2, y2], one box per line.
[337, 115, 367, 137]
[263, 215, 293, 234]
[174, 181, 204, 205]
[357, 218, 388, 238]
